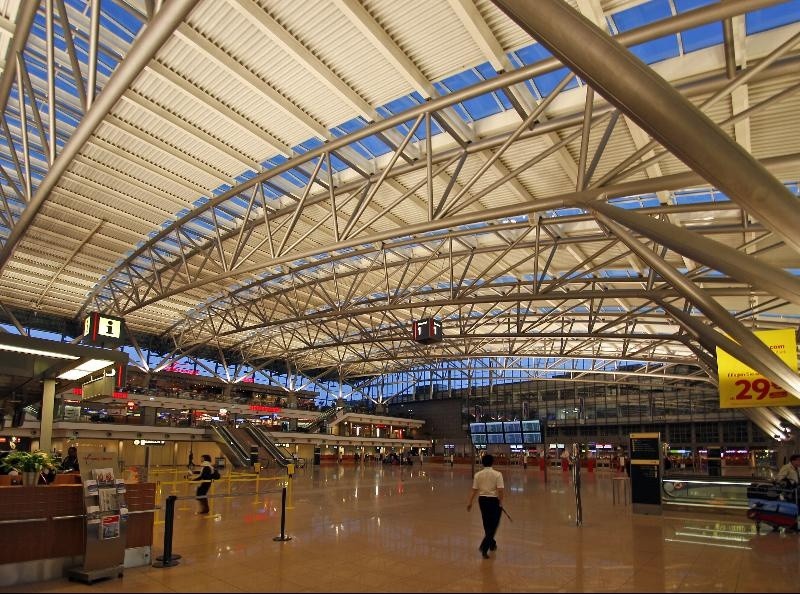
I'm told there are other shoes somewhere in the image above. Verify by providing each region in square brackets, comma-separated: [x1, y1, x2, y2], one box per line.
[772, 528, 780, 533]
[479, 547, 490, 558]
[490, 545, 497, 551]
[785, 529, 796, 534]
[201, 510, 210, 514]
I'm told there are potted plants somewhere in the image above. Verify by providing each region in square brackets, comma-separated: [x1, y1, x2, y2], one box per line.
[0, 450, 55, 486]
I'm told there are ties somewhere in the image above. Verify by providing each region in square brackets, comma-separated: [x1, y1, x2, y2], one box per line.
[795, 467, 800, 482]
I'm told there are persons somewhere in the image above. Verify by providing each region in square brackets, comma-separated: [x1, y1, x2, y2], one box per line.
[59, 447, 79, 471]
[773, 454, 800, 533]
[523, 453, 528, 469]
[339, 451, 411, 466]
[189, 455, 213, 514]
[467, 455, 505, 560]
